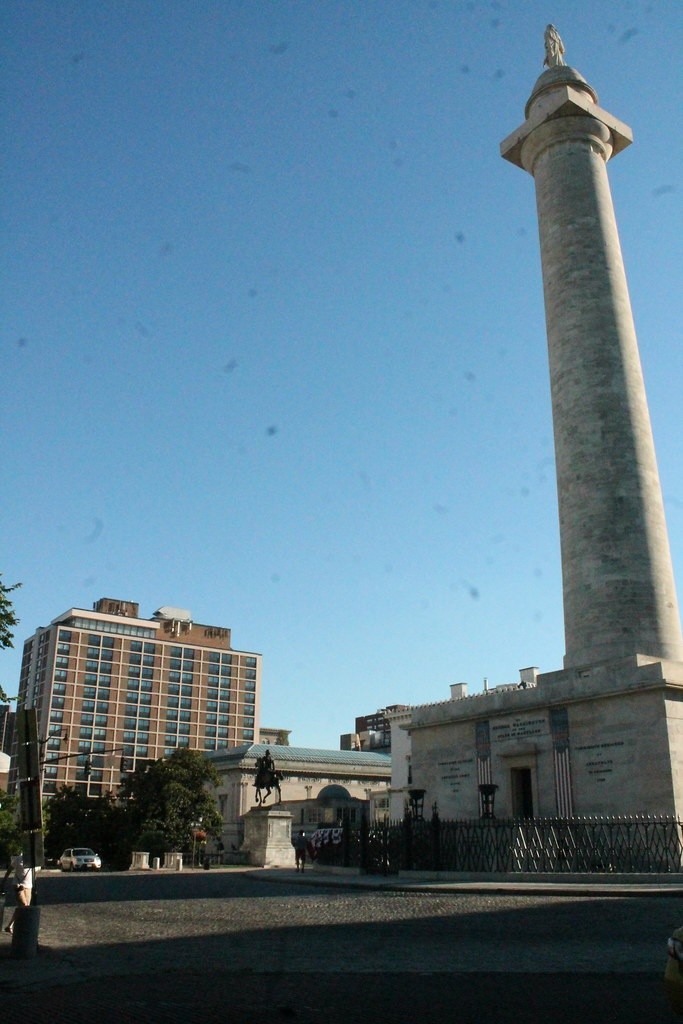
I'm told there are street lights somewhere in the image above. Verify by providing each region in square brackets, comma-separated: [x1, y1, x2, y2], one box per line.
[37, 727, 70, 774]
[187, 811, 202, 871]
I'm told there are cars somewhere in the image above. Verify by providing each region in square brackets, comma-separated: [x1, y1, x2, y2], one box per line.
[59, 848, 102, 871]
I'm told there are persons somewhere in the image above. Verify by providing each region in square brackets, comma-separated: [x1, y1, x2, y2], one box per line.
[251, 749, 275, 787]
[3, 853, 42, 934]
[291, 829, 310, 874]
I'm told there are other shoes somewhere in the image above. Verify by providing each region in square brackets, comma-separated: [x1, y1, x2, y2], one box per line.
[5, 926, 13, 934]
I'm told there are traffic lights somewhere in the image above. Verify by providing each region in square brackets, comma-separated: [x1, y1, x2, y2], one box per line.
[120, 757, 129, 773]
[85, 760, 92, 774]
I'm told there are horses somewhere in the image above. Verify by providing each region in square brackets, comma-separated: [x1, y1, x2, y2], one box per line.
[254, 757, 281, 807]
